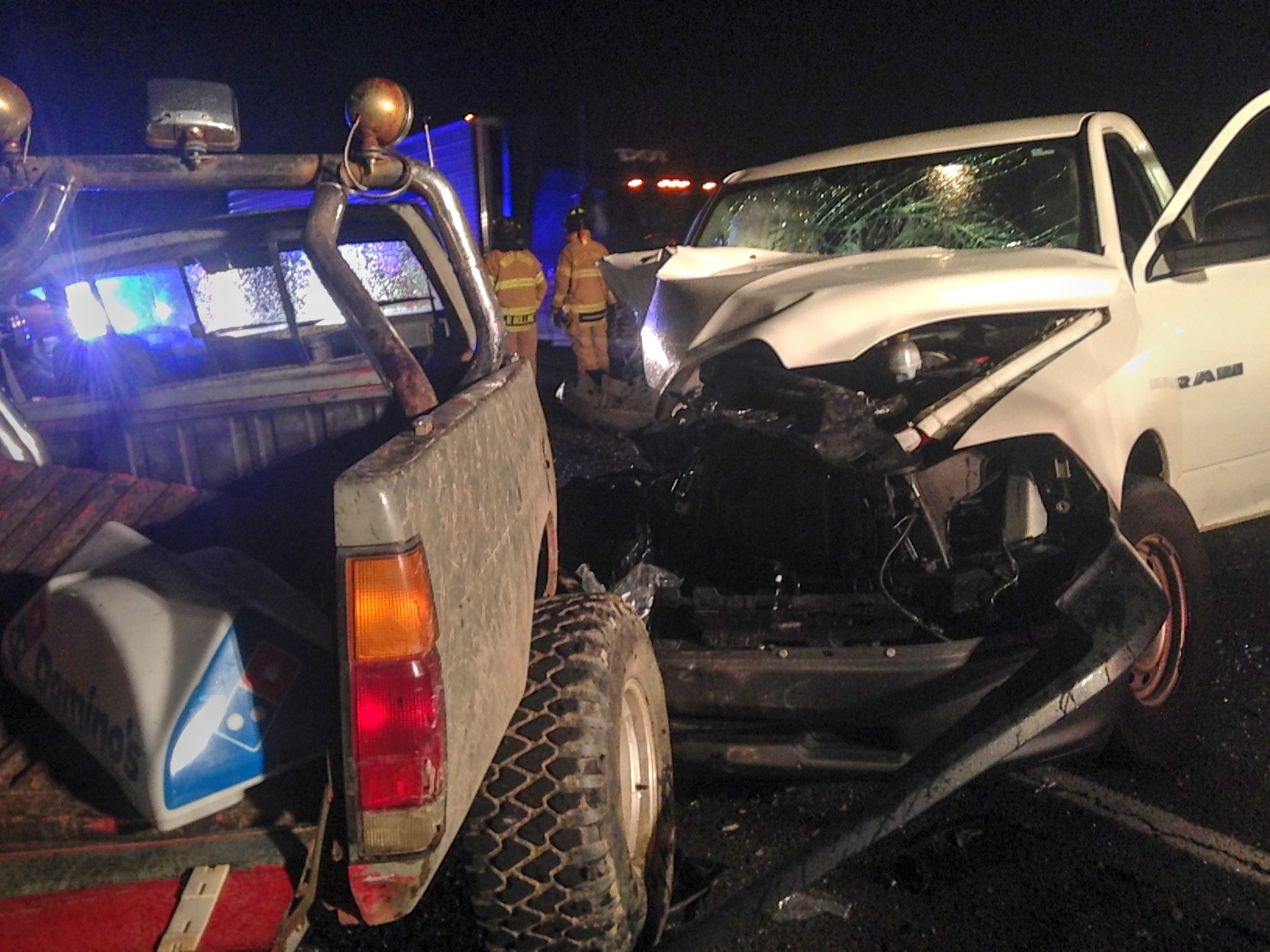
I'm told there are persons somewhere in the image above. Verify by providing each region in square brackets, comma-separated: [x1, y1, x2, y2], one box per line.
[484, 216, 548, 378]
[551, 206, 619, 385]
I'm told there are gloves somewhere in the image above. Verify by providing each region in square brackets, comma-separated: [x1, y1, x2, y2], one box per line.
[553, 313, 561, 329]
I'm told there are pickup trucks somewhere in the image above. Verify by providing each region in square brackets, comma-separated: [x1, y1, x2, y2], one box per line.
[0, 73, 680, 952]
[639, 88, 1270, 759]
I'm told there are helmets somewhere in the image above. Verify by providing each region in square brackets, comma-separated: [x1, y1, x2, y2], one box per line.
[564, 207, 593, 226]
[492, 217, 522, 234]
